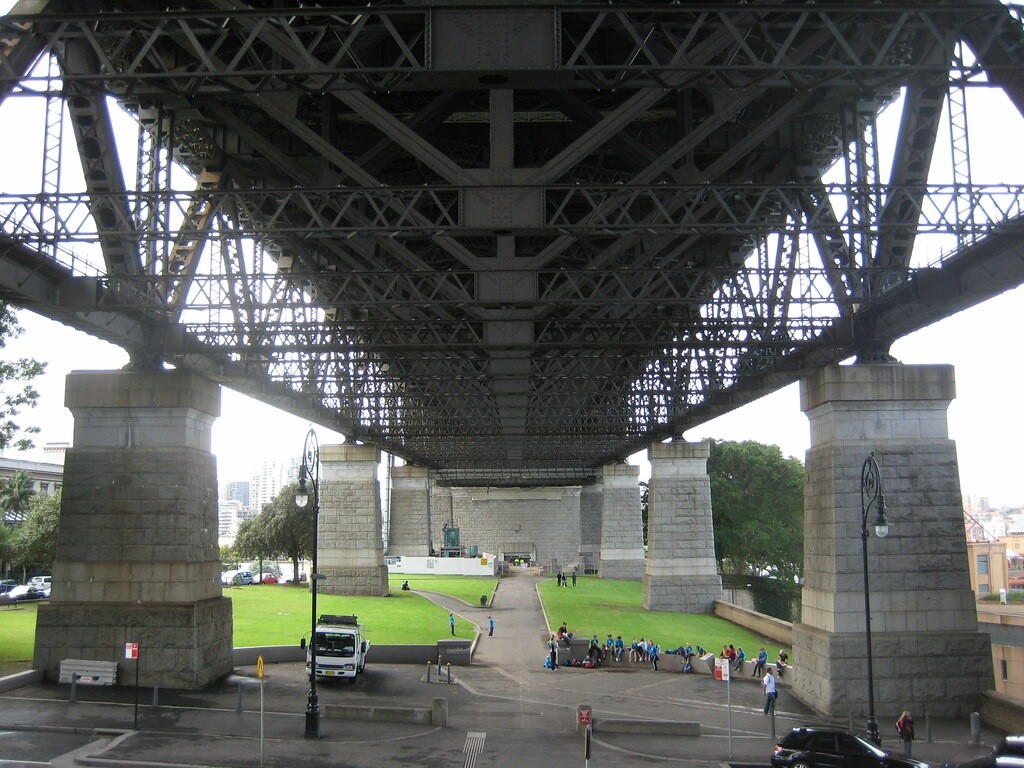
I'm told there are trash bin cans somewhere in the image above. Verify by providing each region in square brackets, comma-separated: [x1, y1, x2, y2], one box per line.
[539, 569, 544, 576]
[999, 589, 1007, 605]
[482, 596, 487, 601]
[300, 573, 306, 581]
[481, 598, 486, 605]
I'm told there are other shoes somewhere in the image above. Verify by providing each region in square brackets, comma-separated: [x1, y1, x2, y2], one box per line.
[737, 668, 741, 671]
[756, 676, 761, 678]
[765, 712, 770, 715]
[733, 668, 737, 671]
[751, 674, 755, 676]
[777, 676, 781, 679]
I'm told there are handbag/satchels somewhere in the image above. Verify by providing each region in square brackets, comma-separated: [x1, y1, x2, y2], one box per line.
[774, 690, 778, 699]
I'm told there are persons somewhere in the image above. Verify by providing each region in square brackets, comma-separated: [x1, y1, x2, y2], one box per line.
[487, 615, 498, 639]
[582, 654, 594, 667]
[571, 572, 577, 587]
[775, 649, 788, 679]
[547, 621, 570, 670]
[589, 634, 624, 662]
[763, 667, 775, 715]
[401, 581, 410, 590]
[719, 644, 745, 671]
[449, 611, 456, 637]
[626, 637, 658, 670]
[750, 647, 768, 678]
[896, 711, 914, 756]
[677, 642, 706, 664]
[556, 572, 568, 587]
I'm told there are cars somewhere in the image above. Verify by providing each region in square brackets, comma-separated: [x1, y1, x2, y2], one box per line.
[944, 736, 1024, 768]
[253, 573, 279, 585]
[221, 570, 254, 586]
[0, 576, 52, 602]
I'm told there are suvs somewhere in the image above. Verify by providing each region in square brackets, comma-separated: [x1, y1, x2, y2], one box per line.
[771, 728, 930, 768]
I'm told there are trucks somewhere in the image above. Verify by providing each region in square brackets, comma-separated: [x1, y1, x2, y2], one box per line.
[300, 614, 371, 685]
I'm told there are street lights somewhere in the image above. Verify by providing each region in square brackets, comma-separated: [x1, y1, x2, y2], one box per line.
[860, 450, 895, 749]
[293, 428, 321, 741]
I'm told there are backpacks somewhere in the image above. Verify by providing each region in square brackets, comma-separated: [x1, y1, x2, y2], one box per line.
[896, 718, 904, 734]
